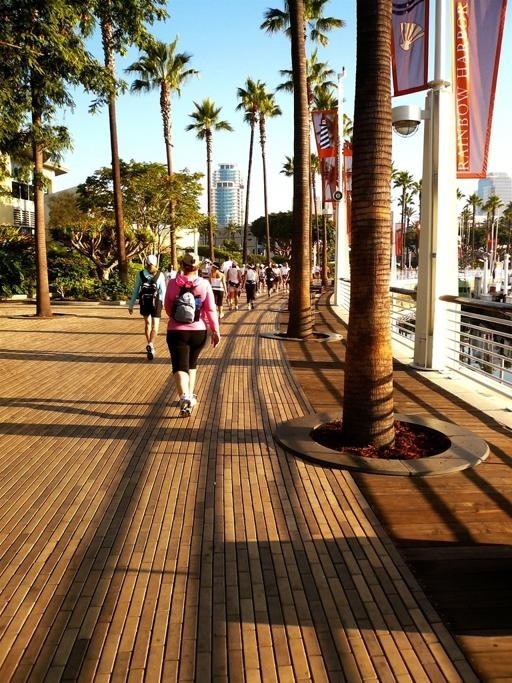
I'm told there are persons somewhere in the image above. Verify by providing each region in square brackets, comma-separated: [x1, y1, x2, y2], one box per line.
[127, 254, 167, 362]
[164, 251, 222, 419]
[163, 255, 331, 312]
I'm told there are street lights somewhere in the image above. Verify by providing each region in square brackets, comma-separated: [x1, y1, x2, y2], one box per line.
[392, 105, 461, 369]
[322, 209, 351, 306]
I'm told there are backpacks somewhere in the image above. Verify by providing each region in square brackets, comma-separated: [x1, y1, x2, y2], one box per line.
[169, 284, 203, 324]
[140, 270, 161, 312]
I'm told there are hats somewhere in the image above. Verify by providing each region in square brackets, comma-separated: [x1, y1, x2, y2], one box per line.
[179, 251, 200, 267]
[147, 255, 157, 266]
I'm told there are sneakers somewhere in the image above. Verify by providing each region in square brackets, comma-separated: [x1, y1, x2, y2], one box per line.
[218, 302, 254, 320]
[146, 343, 155, 360]
[179, 395, 196, 418]
